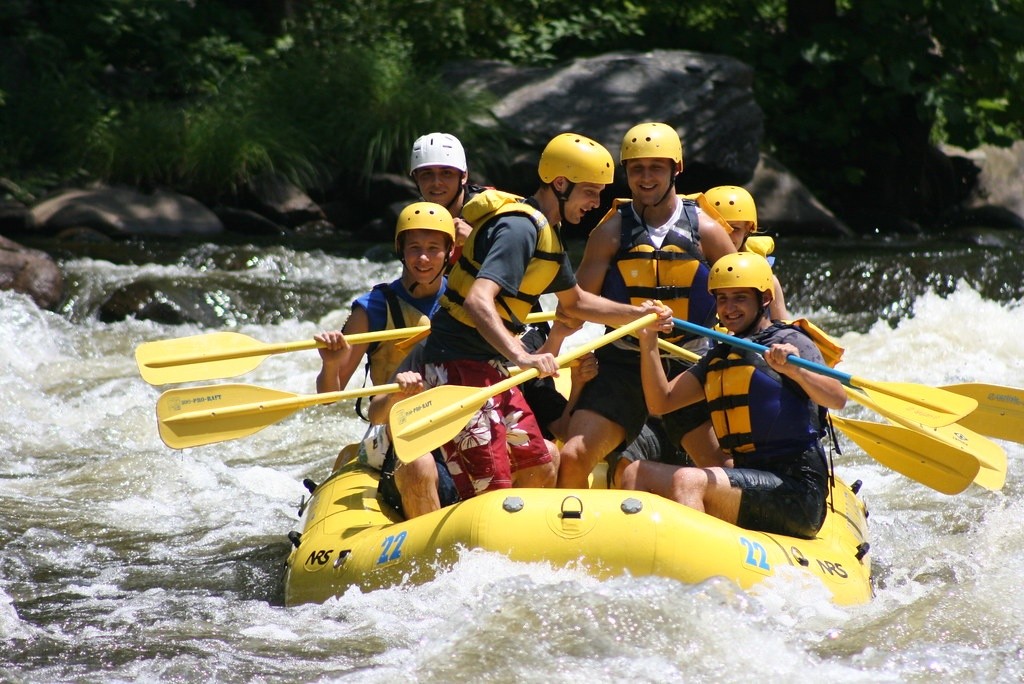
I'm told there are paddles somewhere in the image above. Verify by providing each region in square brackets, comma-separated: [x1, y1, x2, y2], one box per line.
[386, 306, 667, 465]
[156, 357, 588, 449]
[132, 310, 647, 388]
[637, 300, 1024, 496]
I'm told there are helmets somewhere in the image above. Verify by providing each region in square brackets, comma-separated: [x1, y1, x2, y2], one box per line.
[537, 132, 615, 184]
[409, 132, 467, 176]
[619, 122, 684, 173]
[394, 201, 455, 253]
[704, 185, 757, 233]
[707, 251, 775, 300]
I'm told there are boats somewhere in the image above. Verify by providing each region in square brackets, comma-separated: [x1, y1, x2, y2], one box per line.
[283, 440, 880, 625]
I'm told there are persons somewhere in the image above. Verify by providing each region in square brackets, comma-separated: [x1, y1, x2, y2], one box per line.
[612, 253, 847, 538]
[313, 123, 787, 520]
